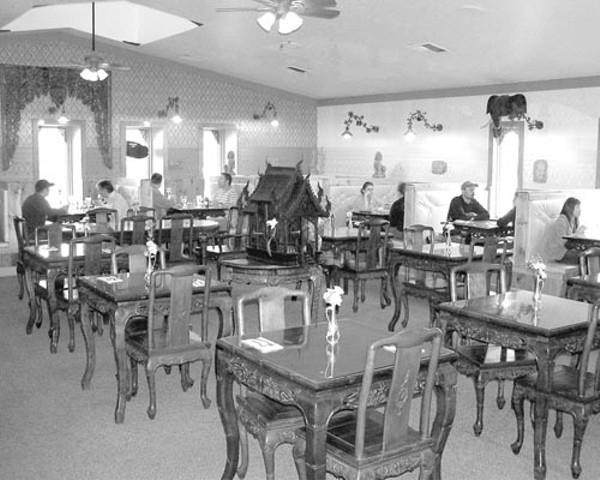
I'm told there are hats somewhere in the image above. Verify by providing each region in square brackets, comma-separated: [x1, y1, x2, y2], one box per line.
[34, 178, 55, 191]
[461, 180, 480, 190]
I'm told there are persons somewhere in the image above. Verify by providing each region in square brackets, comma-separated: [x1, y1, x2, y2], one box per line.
[148, 173, 175, 219]
[93, 179, 129, 220]
[387, 182, 408, 239]
[210, 173, 240, 227]
[346, 182, 383, 228]
[441, 181, 490, 245]
[21, 180, 69, 234]
[544, 198, 588, 277]
[497, 192, 518, 239]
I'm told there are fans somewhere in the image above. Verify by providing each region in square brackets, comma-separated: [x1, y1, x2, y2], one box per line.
[217, 0, 342, 19]
[65, 0, 130, 74]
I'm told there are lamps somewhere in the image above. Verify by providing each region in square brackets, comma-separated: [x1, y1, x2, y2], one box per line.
[158, 96, 187, 126]
[253, 98, 282, 129]
[80, 67, 109, 83]
[256, 10, 305, 36]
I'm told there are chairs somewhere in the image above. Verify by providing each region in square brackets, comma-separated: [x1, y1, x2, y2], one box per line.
[57, 233, 115, 352]
[234, 286, 312, 480]
[429, 237, 508, 327]
[509, 300, 600, 480]
[85, 206, 119, 231]
[12, 214, 36, 299]
[158, 214, 197, 265]
[126, 205, 155, 217]
[31, 223, 78, 329]
[577, 247, 600, 280]
[329, 216, 390, 313]
[122, 263, 212, 421]
[225, 206, 250, 239]
[290, 325, 445, 480]
[118, 216, 156, 249]
[400, 223, 445, 329]
[111, 243, 154, 278]
[445, 262, 537, 438]
